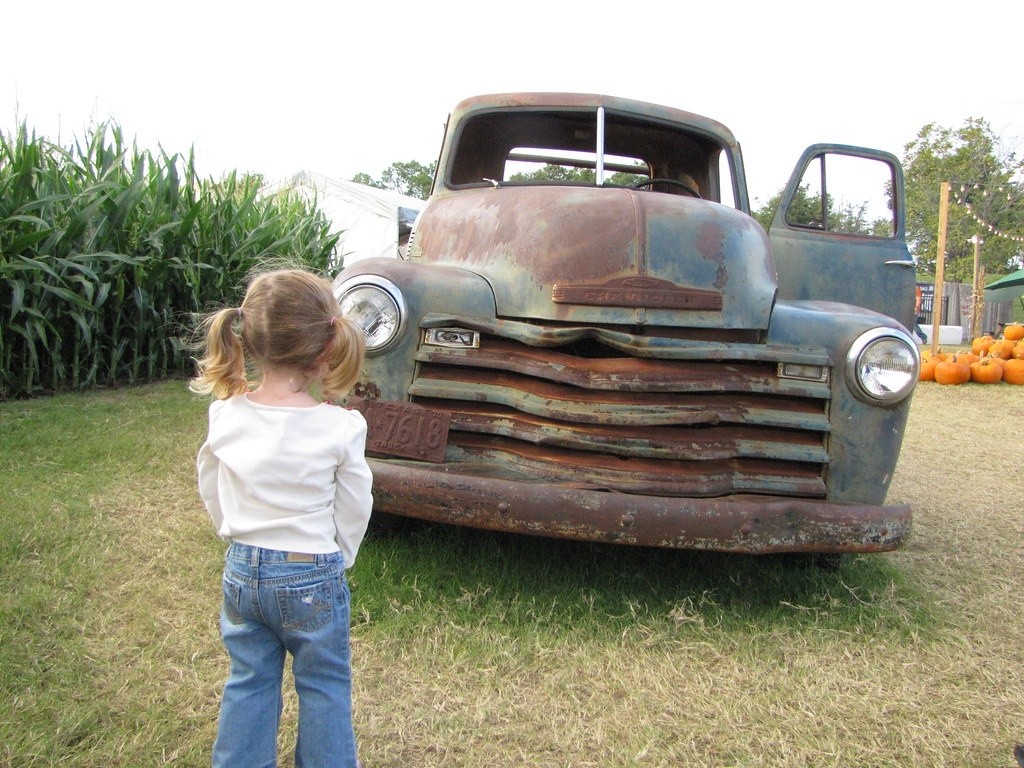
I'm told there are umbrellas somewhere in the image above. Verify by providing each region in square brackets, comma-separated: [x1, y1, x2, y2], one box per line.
[982, 268, 1024, 290]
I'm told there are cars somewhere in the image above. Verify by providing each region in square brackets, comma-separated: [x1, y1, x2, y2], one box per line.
[329, 93, 922, 554]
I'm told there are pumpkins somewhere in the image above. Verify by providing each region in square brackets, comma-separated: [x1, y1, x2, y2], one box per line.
[920, 323, 1024, 385]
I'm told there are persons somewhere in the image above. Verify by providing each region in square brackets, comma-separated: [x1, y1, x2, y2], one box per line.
[181, 257, 374, 768]
[913, 285, 929, 345]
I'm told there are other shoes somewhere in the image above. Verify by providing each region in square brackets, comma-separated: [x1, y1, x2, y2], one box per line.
[922, 338, 927, 344]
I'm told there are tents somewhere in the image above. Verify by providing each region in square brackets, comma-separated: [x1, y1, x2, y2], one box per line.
[250, 169, 427, 268]
